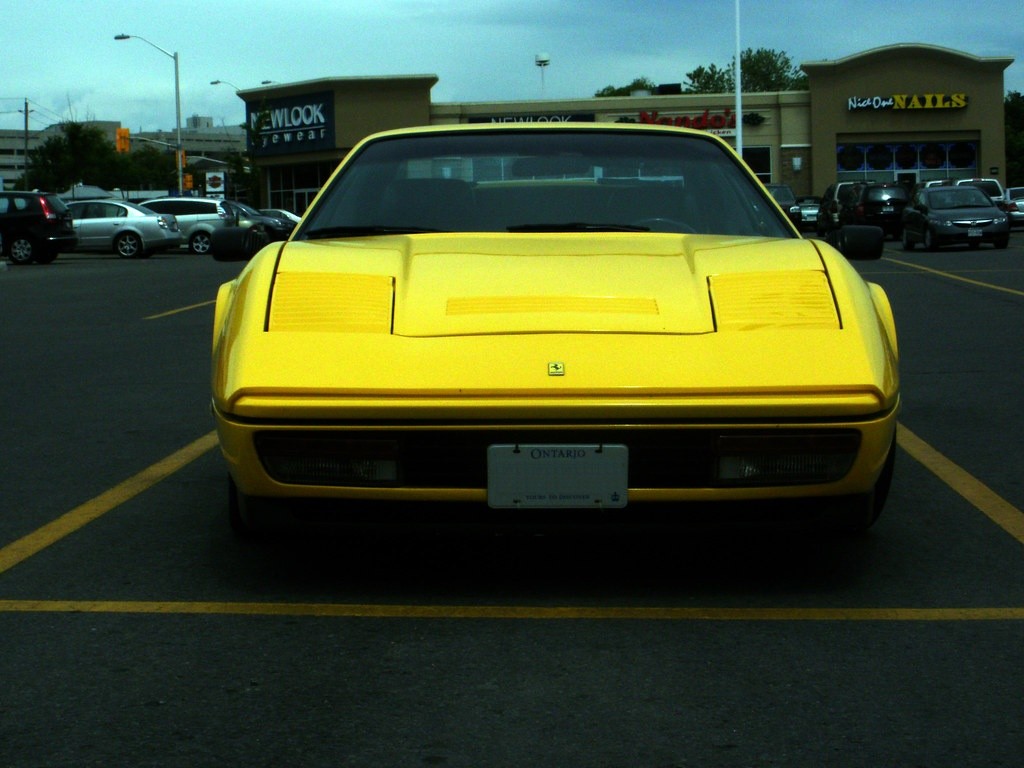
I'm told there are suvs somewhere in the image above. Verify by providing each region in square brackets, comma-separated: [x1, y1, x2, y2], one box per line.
[912, 179, 951, 189]
[838, 181, 911, 240]
[816, 179, 876, 235]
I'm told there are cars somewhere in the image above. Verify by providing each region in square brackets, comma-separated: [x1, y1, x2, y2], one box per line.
[139, 197, 226, 255]
[793, 194, 823, 233]
[62, 198, 181, 259]
[227, 199, 297, 241]
[763, 182, 797, 211]
[0, 191, 79, 265]
[902, 185, 1009, 253]
[1003, 186, 1024, 232]
[260, 208, 302, 224]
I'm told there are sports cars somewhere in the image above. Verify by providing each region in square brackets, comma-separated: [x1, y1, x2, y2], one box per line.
[208, 118, 903, 534]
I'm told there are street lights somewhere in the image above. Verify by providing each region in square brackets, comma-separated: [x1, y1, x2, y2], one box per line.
[114, 34, 183, 196]
[535, 51, 551, 100]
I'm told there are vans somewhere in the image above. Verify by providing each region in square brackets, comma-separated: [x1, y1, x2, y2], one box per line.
[944, 178, 1007, 215]
